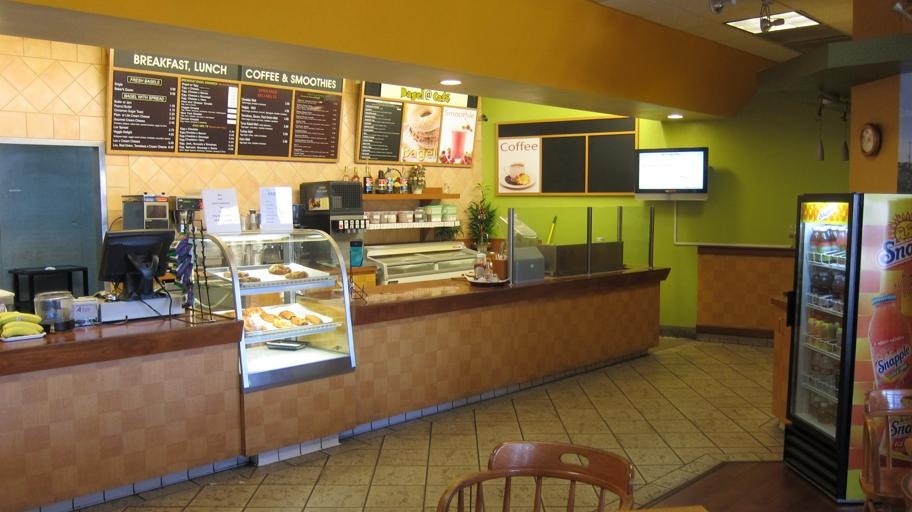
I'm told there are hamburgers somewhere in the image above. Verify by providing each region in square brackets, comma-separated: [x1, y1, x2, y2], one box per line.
[408, 105, 441, 150]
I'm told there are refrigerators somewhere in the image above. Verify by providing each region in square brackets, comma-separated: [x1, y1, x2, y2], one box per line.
[782, 192, 912, 506]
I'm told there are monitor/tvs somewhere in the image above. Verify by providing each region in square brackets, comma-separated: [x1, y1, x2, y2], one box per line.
[634, 146, 709, 203]
[98, 232, 173, 299]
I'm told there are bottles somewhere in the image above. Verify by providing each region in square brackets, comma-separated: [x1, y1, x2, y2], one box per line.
[809, 268, 845, 302]
[473, 253, 493, 281]
[33, 290, 76, 334]
[809, 225, 848, 262]
[807, 392, 836, 428]
[241, 208, 261, 231]
[342, 165, 408, 196]
[349, 241, 363, 267]
[868, 294, 912, 389]
[808, 351, 838, 396]
[804, 308, 843, 357]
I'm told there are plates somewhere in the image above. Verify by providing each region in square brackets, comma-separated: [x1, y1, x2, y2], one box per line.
[463, 272, 511, 284]
[500, 174, 535, 189]
[0, 332, 47, 342]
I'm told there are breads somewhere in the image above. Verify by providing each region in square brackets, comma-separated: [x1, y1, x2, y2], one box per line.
[284, 271, 308, 279]
[268, 263, 291, 275]
[223, 268, 260, 283]
[224, 307, 322, 331]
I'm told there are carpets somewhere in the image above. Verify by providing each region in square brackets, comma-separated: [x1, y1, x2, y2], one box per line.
[636, 461, 841, 512]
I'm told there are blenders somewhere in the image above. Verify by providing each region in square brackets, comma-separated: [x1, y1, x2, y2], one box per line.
[293, 204, 305, 260]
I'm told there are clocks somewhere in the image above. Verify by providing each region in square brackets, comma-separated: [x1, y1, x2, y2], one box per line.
[860, 125, 880, 157]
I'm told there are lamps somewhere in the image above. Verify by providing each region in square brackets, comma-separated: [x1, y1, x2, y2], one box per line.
[759, 0, 785, 33]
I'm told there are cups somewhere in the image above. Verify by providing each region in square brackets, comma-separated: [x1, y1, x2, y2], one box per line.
[494, 260, 507, 280]
[504, 163, 525, 182]
[452, 130, 467, 159]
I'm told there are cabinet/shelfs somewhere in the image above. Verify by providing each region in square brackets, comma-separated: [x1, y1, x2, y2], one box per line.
[363, 187, 464, 258]
[8, 264, 89, 311]
[190, 228, 357, 392]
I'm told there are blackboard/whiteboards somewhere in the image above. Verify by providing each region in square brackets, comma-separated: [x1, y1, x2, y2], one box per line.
[0, 136, 108, 301]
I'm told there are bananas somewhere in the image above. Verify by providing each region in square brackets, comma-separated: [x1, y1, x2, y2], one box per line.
[0, 312, 44, 337]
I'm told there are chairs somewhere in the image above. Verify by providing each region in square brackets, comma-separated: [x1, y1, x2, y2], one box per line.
[436, 442, 635, 512]
